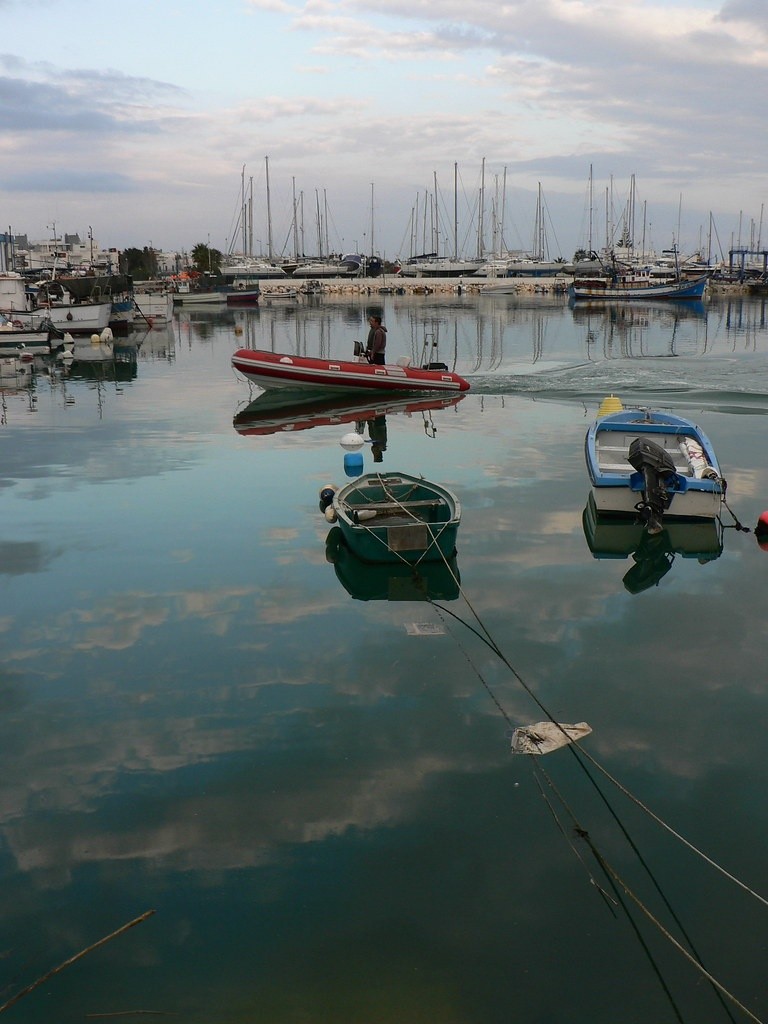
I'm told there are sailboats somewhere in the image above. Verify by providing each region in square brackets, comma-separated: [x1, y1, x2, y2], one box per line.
[215, 156, 768, 291]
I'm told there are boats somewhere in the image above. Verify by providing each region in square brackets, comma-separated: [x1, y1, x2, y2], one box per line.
[569, 262, 708, 300]
[232, 390, 467, 437]
[231, 349, 470, 396]
[319, 471, 461, 561]
[325, 526, 461, 601]
[584, 393, 724, 519]
[0, 301, 52, 357]
[581, 491, 724, 565]
[0, 222, 261, 331]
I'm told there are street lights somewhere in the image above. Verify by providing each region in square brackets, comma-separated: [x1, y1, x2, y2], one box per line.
[87, 226, 94, 264]
[46, 223, 59, 258]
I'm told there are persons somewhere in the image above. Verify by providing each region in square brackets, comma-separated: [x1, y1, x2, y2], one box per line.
[366, 315, 388, 364]
